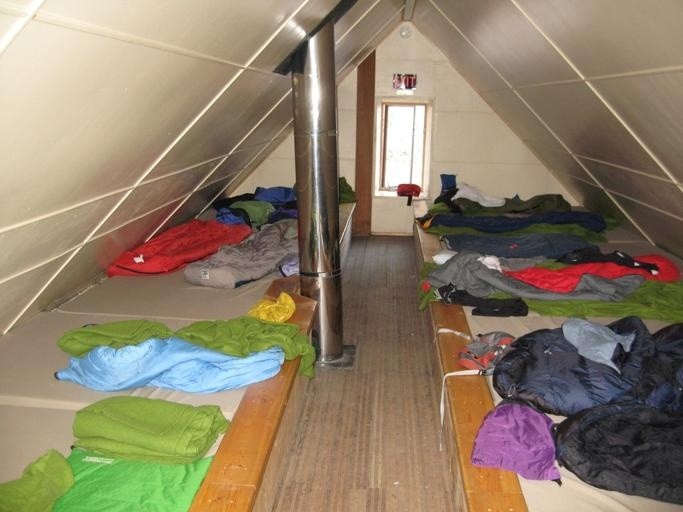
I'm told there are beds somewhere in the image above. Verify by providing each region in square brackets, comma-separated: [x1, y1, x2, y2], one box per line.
[0, 202, 357, 511]
[411, 201, 683, 512]
